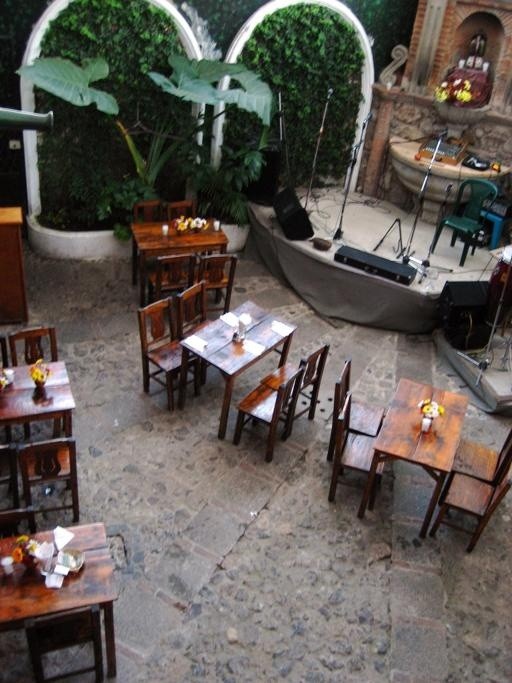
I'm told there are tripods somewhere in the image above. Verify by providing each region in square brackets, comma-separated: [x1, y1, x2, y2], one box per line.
[390, 190, 428, 277]
[412, 191, 454, 282]
[457, 302, 494, 386]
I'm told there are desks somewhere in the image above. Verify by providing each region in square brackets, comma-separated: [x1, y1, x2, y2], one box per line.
[130, 216, 230, 305]
[1, 361, 76, 437]
[181, 301, 298, 439]
[0, 207, 29, 324]
[357, 377, 471, 539]
[0, 522, 117, 682]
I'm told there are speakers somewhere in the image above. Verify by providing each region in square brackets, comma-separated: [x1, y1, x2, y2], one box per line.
[440, 281, 493, 348]
[276, 186, 317, 240]
[335, 245, 415, 285]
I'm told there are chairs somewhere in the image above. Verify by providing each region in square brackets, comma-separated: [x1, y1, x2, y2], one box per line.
[260, 345, 332, 438]
[335, 355, 386, 437]
[146, 252, 199, 323]
[8, 326, 70, 441]
[130, 200, 165, 222]
[1, 443, 26, 537]
[429, 175, 499, 266]
[429, 468, 511, 553]
[24, 604, 102, 682]
[174, 280, 215, 386]
[437, 428, 512, 505]
[162, 200, 199, 221]
[19, 439, 79, 533]
[191, 254, 240, 315]
[0, 335, 8, 368]
[327, 392, 387, 512]
[136, 297, 203, 413]
[232, 369, 305, 464]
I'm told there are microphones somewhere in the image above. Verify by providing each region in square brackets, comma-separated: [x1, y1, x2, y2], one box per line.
[438, 130, 448, 138]
[326, 89, 333, 100]
[363, 113, 372, 126]
